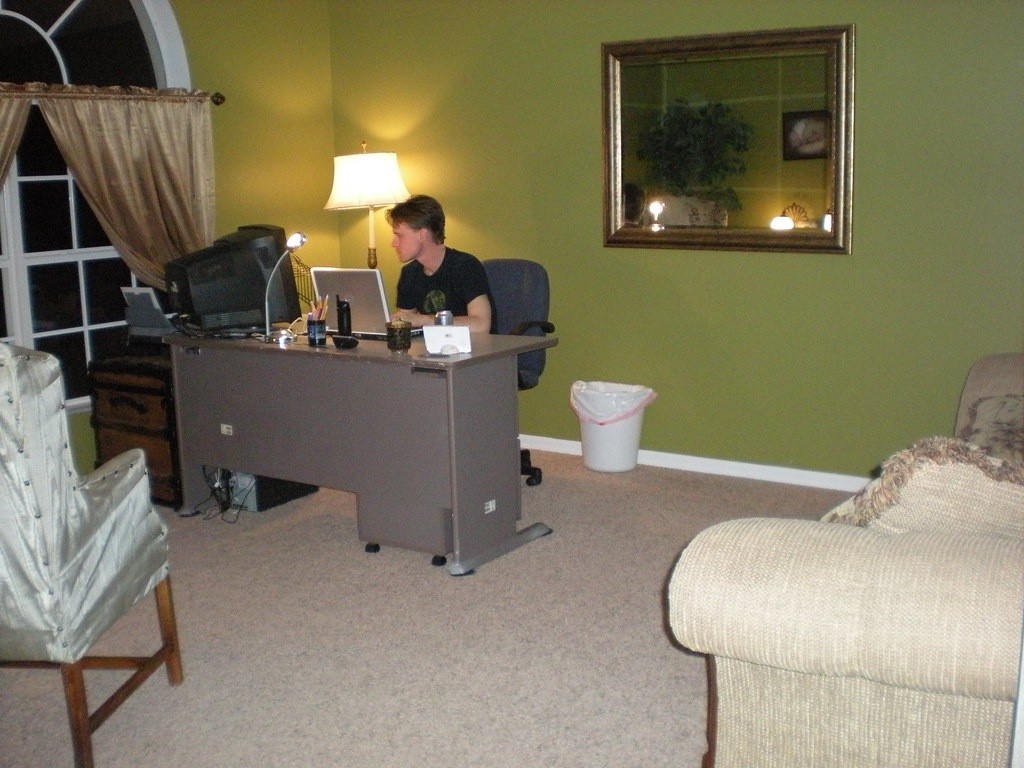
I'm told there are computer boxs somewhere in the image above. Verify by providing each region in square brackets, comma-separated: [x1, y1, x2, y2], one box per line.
[224, 471, 318, 514]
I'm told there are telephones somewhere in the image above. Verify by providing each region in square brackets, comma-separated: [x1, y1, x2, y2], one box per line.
[330, 293, 360, 349]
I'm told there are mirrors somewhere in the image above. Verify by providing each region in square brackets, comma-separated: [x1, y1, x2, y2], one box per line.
[600, 21, 856, 255]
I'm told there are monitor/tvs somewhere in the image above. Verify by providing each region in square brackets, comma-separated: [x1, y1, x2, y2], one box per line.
[165, 223, 302, 334]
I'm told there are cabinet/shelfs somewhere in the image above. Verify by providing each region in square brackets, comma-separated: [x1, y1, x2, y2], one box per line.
[79, 347, 184, 513]
[161, 314, 560, 576]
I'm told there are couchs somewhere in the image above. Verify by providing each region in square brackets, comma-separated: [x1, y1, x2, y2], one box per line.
[667, 351, 1024, 768]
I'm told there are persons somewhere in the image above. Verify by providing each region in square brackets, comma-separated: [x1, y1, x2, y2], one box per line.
[384, 192, 499, 335]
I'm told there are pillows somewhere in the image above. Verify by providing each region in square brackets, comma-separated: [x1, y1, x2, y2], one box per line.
[815, 433, 1023, 546]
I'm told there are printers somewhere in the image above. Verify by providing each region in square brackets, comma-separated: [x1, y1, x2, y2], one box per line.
[125, 305, 185, 368]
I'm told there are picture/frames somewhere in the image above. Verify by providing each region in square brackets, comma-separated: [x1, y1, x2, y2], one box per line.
[782, 110, 833, 161]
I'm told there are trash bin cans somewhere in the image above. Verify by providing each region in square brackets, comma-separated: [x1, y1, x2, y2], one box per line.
[570, 380, 653, 472]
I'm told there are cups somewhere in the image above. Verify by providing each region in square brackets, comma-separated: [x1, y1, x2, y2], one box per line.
[385, 317, 412, 353]
[307, 320, 328, 347]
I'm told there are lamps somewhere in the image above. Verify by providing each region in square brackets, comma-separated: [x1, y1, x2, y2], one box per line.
[322, 138, 413, 270]
[257, 232, 313, 345]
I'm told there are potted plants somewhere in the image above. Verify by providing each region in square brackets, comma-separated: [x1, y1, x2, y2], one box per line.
[634, 94, 758, 228]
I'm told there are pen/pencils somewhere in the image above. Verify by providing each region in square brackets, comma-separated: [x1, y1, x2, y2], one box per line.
[306, 294, 329, 321]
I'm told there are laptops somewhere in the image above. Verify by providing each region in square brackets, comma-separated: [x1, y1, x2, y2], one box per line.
[311, 268, 423, 340]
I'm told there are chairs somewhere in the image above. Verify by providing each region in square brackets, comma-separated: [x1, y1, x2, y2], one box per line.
[479, 258, 555, 487]
[0, 341, 186, 768]
